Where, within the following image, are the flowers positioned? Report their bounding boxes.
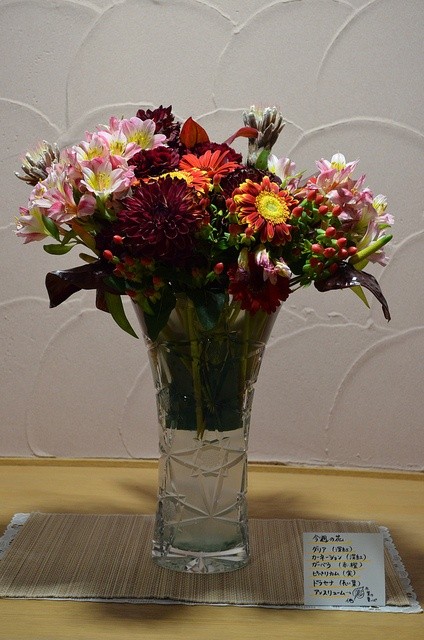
[14,104,393,432]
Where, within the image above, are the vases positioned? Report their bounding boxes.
[126,288,293,573]
